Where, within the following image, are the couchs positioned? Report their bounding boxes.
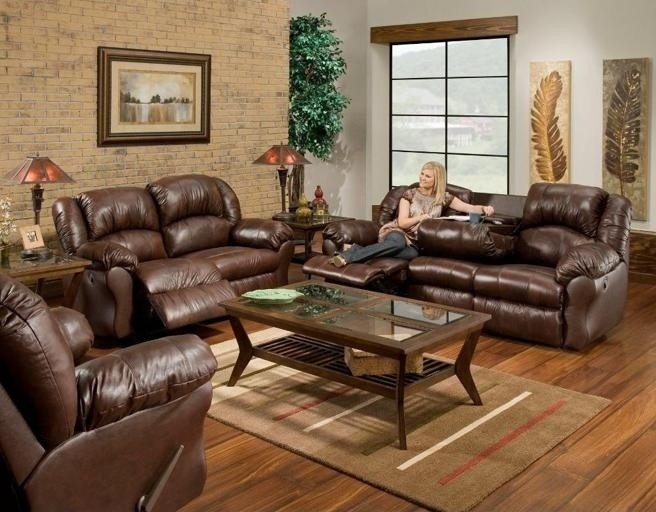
[50,173,297,343]
[301,180,633,354]
[0,272,219,512]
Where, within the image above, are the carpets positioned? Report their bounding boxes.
[204,325,615,511]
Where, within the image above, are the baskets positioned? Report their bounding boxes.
[344,334,424,376]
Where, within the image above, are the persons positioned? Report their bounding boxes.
[329,160,496,268]
[30,231,38,241]
[27,232,32,240]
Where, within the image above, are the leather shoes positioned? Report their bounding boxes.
[329,256,345,267]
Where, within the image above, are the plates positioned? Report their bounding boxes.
[240,287,306,305]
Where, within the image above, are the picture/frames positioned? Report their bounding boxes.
[96,45,212,147]
[20,224,45,250]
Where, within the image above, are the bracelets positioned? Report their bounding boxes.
[481,206,487,213]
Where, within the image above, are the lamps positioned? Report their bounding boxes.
[2,151,76,263]
[252,140,312,222]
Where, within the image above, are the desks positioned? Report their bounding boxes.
[289,215,355,265]
[0,249,93,298]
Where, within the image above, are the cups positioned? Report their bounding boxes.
[468,212,484,225]
